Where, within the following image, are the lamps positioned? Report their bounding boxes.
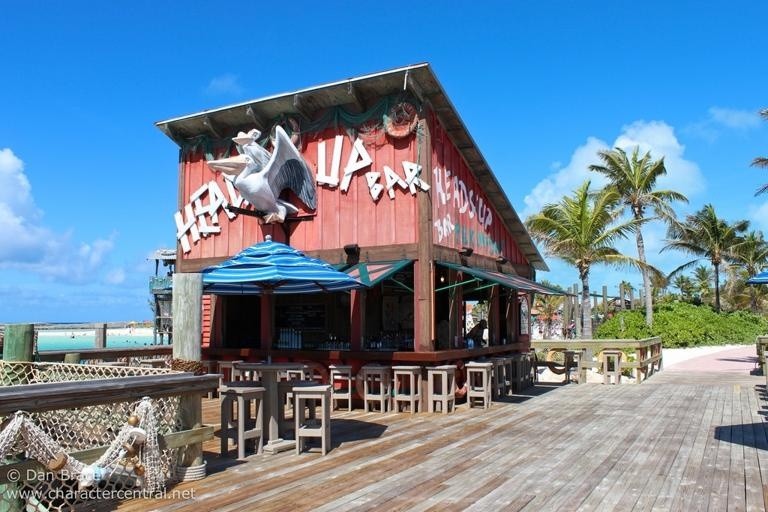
[459,247,507,265]
[344,243,360,257]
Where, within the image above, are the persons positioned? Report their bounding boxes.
[465,318,489,348]
[436,308,457,350]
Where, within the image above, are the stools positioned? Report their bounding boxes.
[97,350,622,460]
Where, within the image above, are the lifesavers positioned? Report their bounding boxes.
[298,362,329,406]
[454,360,467,399]
[546,348,573,374]
[599,348,627,375]
[383,102,418,137]
[356,363,399,402]
[270,116,301,147]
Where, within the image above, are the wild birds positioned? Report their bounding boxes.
[206,125,318,223]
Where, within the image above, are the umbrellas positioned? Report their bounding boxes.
[744,270,768,286]
[199,233,371,365]
